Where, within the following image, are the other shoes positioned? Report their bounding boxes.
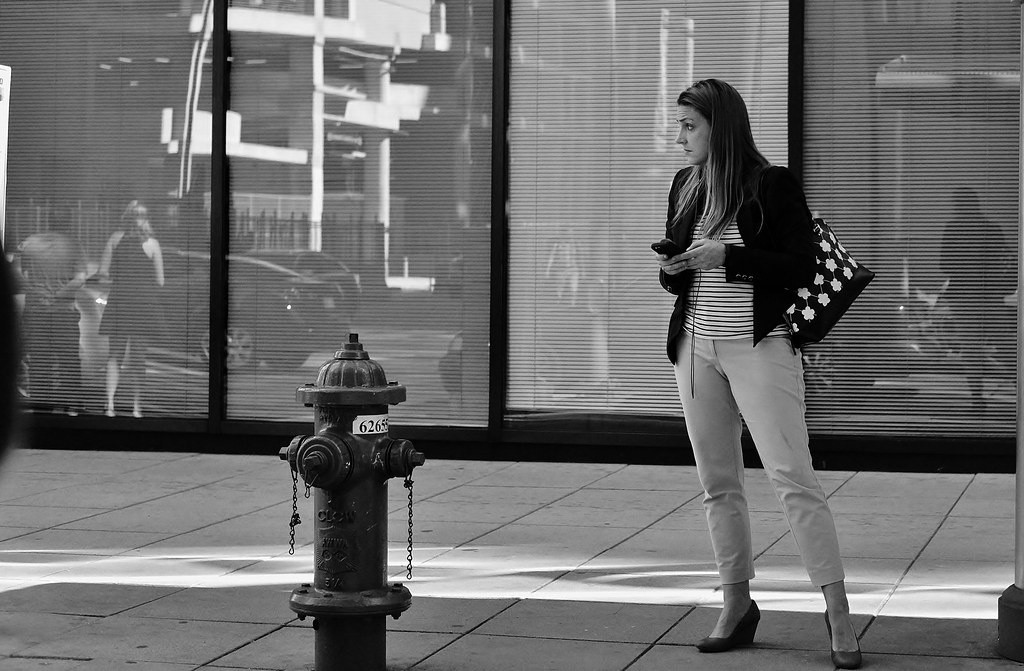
[105,411,116,416]
[964,406,987,420]
[133,411,144,417]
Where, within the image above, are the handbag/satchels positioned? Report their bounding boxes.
[1000,255,1018,296]
[782,219,875,347]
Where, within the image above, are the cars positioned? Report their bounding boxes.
[4,249,362,405]
[806,290,915,408]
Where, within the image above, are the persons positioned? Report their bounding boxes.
[545,226,585,307]
[97,200,165,417]
[940,186,1017,416]
[12,203,89,409]
[654,78,862,670]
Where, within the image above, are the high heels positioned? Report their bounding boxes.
[824,610,863,669]
[696,599,761,649]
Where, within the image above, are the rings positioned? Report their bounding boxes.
[661,267,665,272]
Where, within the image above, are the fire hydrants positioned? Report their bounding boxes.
[279,334,425,671]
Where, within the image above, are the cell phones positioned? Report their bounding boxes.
[650,241,686,258]
